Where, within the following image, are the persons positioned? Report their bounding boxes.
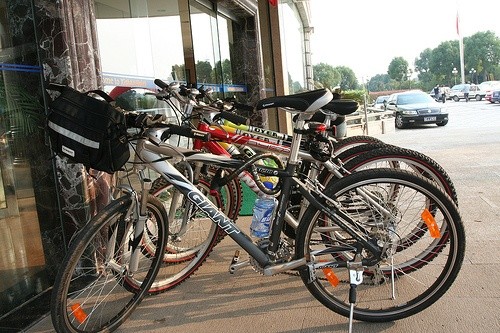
[461,81,469,102]
[440,85,447,103]
[434,84,440,102]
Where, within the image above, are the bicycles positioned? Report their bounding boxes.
[44,81,466,333]
[111,77,460,296]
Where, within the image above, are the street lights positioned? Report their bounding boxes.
[452,68,458,85]
[468,67,477,83]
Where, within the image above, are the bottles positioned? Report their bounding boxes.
[249,181,276,238]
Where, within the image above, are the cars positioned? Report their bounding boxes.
[448,81,500,104]
[430,86,452,101]
[386,91,448,129]
[373,93,399,117]
